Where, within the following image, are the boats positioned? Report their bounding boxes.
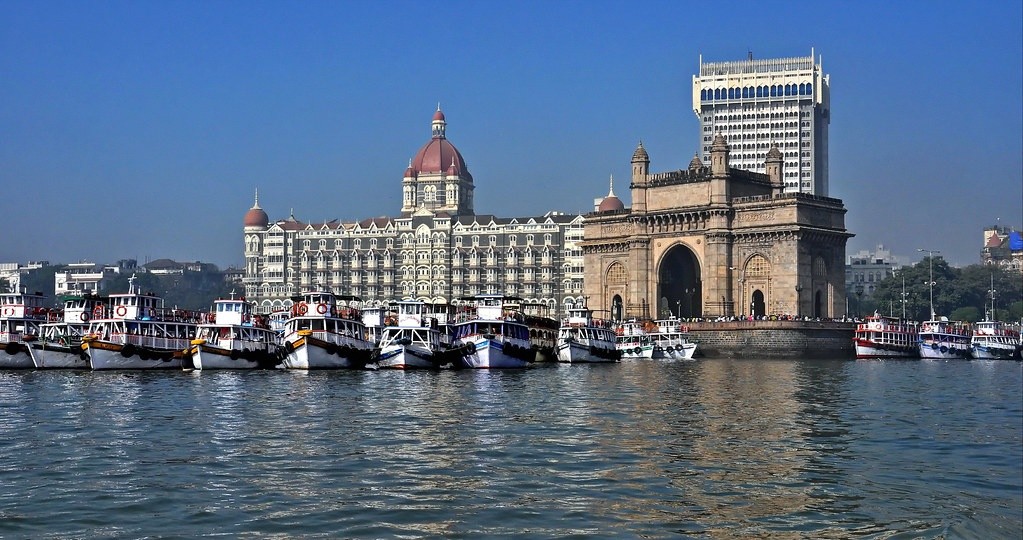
[851,276,919,359]
[276,292,375,370]
[970,273,1023,361]
[522,303,561,363]
[190,287,277,370]
[453,294,537,370]
[81,272,200,371]
[554,308,618,363]
[613,314,698,361]
[375,301,442,370]
[22,294,112,369]
[917,282,972,359]
[0,293,64,371]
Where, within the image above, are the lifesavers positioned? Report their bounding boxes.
[502,342,535,363]
[326,342,374,364]
[873,343,917,354]
[627,349,632,354]
[38,307,47,318]
[298,304,308,315]
[5,342,19,355]
[675,344,683,351]
[49,308,56,321]
[138,348,174,362]
[665,346,673,354]
[990,349,998,356]
[657,347,663,352]
[4,307,15,316]
[229,340,293,365]
[931,343,966,357]
[859,323,863,329]
[924,325,932,332]
[634,347,642,354]
[116,306,127,316]
[999,348,1013,356]
[434,341,476,366]
[330,305,337,316]
[24,308,35,319]
[875,323,882,330]
[317,303,328,314]
[121,343,136,358]
[589,346,624,361]
[80,312,90,321]
[242,313,251,324]
[93,306,103,316]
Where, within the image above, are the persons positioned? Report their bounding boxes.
[683,314,859,323]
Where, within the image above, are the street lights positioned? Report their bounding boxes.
[915,247,941,321]
[686,288,695,322]
